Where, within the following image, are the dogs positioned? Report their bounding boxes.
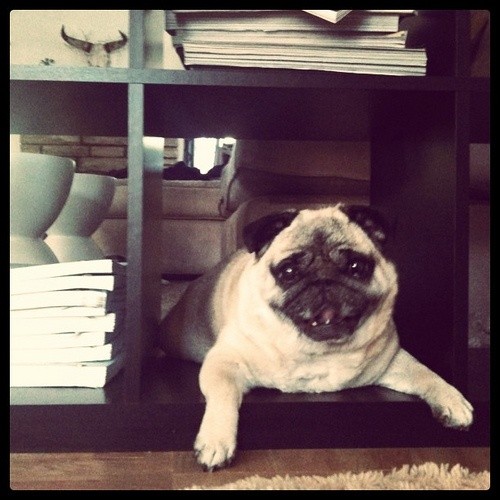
[156,203,473,471]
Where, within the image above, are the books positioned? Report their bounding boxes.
[163,10,431,77]
[8,257,127,391]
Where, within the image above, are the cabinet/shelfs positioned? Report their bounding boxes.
[10,7,490,452]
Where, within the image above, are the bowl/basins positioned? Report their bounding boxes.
[7,153,78,265]
[44,173,120,268]
